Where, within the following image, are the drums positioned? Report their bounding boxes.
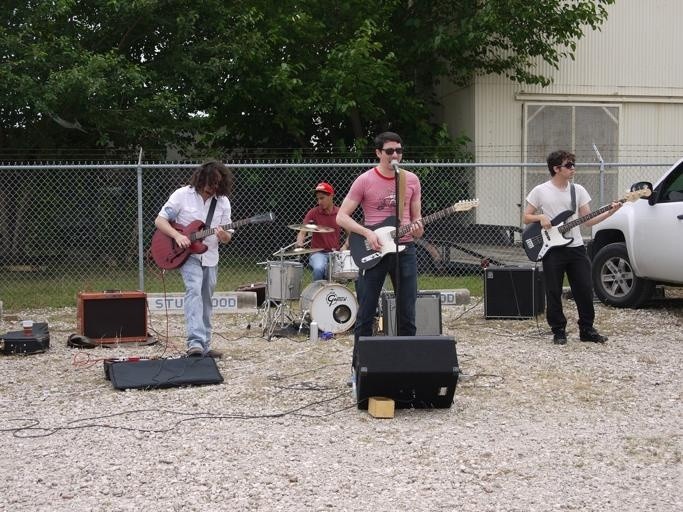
[264,260,303,301]
[299,280,357,335]
[326,251,361,280]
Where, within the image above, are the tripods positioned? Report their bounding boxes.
[263,249,309,341]
[248,271,297,328]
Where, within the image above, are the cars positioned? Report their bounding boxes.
[413,223,544,275]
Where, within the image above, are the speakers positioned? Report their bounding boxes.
[349,336,460,409]
[485,265,546,318]
[78,290,148,343]
[382,291,442,336]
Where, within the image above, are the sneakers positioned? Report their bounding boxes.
[187,347,223,357]
[555,330,567,344]
[581,332,608,343]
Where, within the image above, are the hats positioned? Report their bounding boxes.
[310,181,335,197]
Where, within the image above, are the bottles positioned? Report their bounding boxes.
[309,319,318,345]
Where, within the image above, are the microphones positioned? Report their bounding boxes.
[391,159,401,174]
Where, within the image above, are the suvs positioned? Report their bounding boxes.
[586,158,682,310]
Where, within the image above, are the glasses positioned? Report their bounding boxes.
[380,147,403,155]
[555,162,575,169]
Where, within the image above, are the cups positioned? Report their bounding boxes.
[22,320,33,336]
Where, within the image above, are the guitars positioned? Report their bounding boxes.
[523,185,652,261]
[151,212,275,270]
[349,199,480,270]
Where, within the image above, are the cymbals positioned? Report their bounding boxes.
[276,247,323,257]
[288,224,334,232]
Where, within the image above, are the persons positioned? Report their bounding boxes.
[294,181,356,282]
[334,130,426,375]
[520,150,623,347]
[153,160,236,358]
